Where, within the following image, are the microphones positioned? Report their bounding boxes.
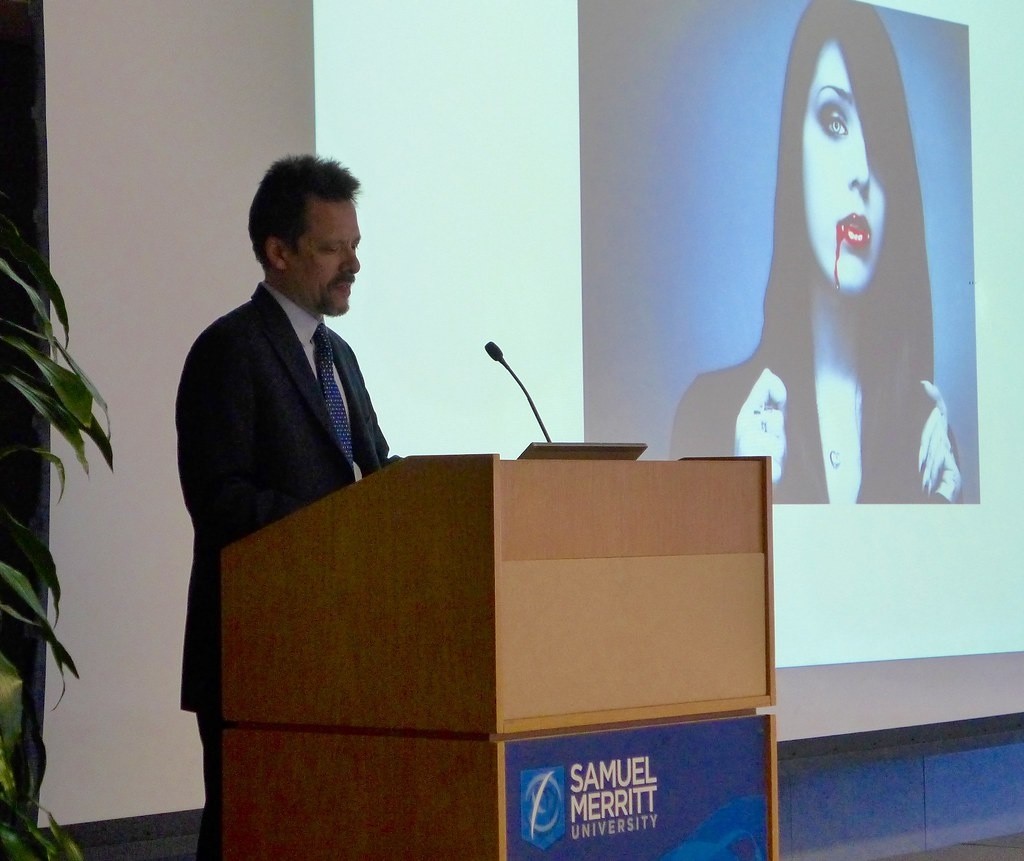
[485,341,552,443]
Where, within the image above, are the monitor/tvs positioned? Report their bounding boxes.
[515,443,646,460]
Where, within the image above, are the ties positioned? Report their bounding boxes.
[313,323,353,470]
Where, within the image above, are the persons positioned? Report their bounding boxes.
[668,1,974,504]
[171,154,400,859]
